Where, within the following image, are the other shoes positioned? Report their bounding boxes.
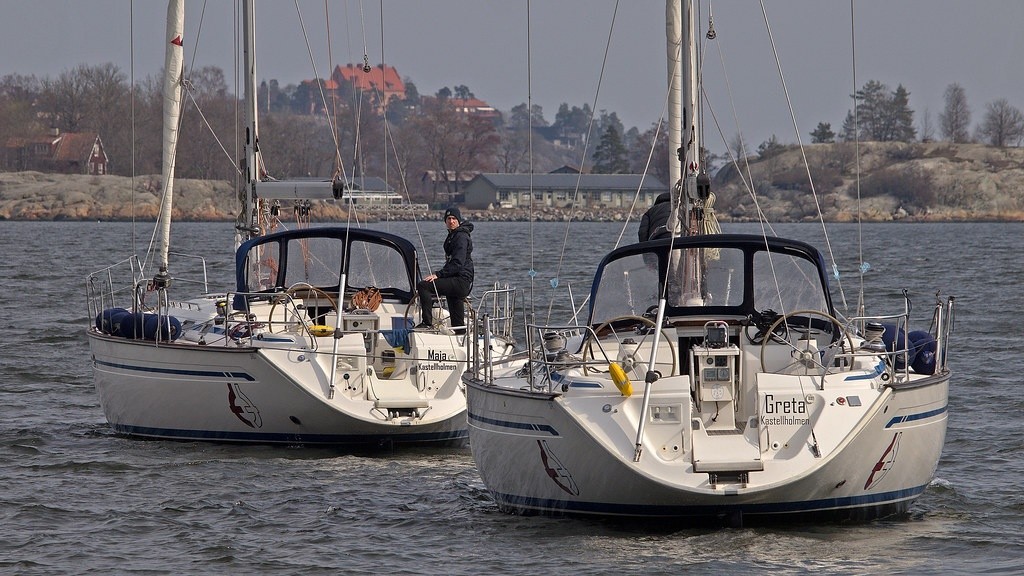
[415,322,433,329]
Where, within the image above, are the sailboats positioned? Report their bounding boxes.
[79,1,521,446]
[460,0,956,528]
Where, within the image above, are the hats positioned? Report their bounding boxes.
[444,209,461,224]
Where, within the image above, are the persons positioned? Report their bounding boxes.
[637,192,673,308]
[415,207,474,334]
